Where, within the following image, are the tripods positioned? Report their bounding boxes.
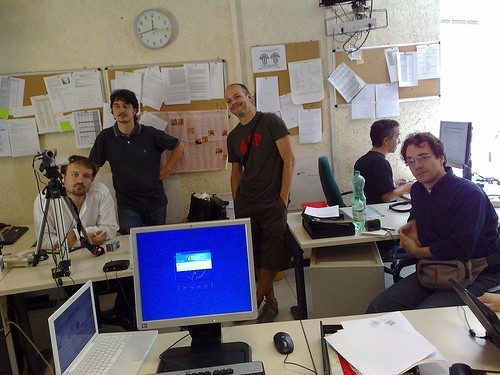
[33,167,99,276]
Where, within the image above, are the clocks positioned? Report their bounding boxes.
[134,9,173,49]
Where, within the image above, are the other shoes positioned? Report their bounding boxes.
[120,295,138,332]
[97,291,127,327]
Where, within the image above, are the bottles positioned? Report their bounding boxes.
[352,196,364,232]
[351,170,366,218]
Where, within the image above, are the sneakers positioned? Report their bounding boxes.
[232,294,265,325]
[258,298,280,324]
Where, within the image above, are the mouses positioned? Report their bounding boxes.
[274,332,294,354]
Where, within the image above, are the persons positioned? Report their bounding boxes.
[33,156,120,250]
[364,131,500,314]
[224,83,295,324]
[476,292,500,312]
[353,119,411,206]
[89,89,184,315]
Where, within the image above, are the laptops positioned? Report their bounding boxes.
[48,280,158,375]
[448,277,500,348]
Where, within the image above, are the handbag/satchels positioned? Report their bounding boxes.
[413,254,500,292]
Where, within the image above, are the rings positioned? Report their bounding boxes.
[94,233,97,236]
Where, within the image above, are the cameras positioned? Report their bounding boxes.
[130,218,258,330]
[440,121,472,168]
[38,147,70,171]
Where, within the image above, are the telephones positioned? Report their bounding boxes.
[3,248,39,269]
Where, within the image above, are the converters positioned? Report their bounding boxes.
[103,260,130,272]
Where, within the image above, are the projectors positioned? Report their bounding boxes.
[335,16,383,31]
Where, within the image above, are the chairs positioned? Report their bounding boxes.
[319,156,347,207]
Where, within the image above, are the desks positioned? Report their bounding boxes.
[138,306,500,375]
[0,225,36,280]
[0,235,134,375]
[287,202,412,320]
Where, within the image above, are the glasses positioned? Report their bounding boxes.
[401,151,435,168]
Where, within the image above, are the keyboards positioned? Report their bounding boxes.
[0,227,28,245]
[147,361,265,375]
